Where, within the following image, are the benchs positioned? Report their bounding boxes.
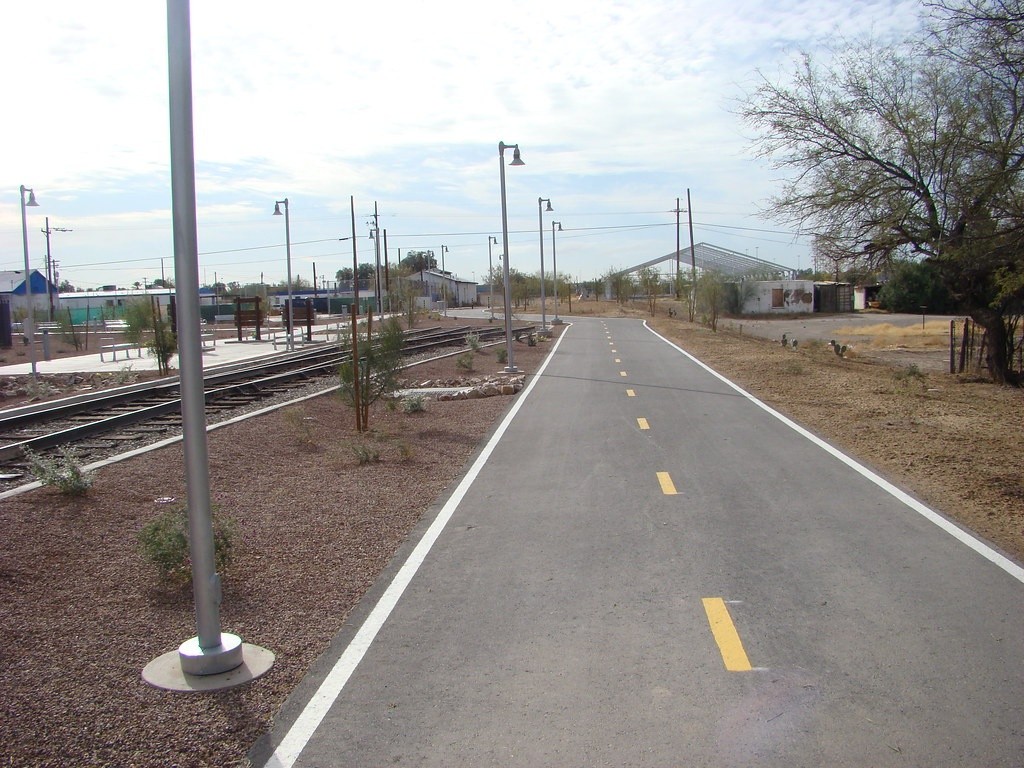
[35,322,63,332]
[104,320,131,334]
[99,338,142,363]
[198,323,215,346]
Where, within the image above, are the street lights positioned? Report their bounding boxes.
[442,244,450,319]
[368,227,385,323]
[551,221,564,321]
[497,140,525,373]
[426,250,435,300]
[538,196,554,330]
[488,235,499,320]
[19,183,40,376]
[272,197,295,347]
